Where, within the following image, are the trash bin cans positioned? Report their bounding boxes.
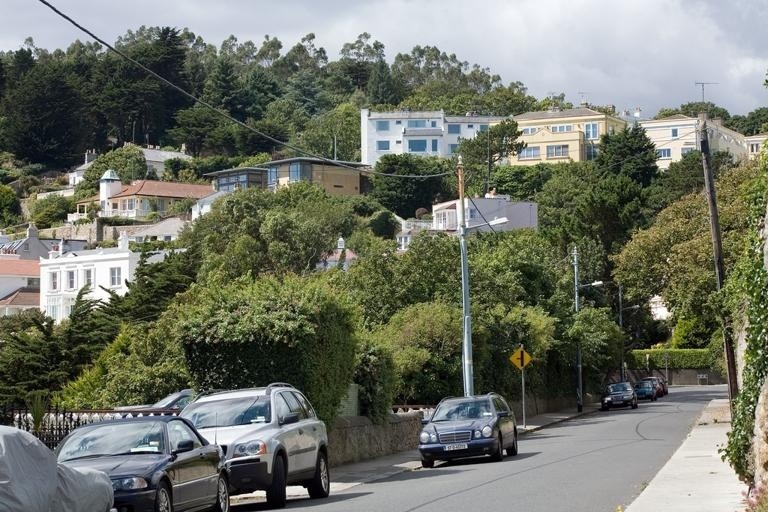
[697,374,708,385]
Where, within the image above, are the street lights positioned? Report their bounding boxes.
[619,305,641,381]
[461,214,511,398]
[575,280,603,412]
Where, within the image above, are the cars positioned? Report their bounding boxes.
[599,375,669,411]
[122,390,198,419]
[52,412,234,511]
[418,390,520,468]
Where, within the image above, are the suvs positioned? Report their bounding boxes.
[179,380,334,509]
